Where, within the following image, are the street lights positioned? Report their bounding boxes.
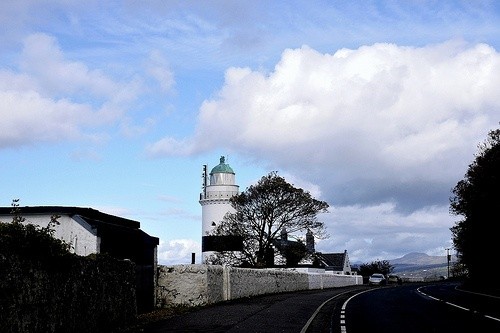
[446,248,452,280]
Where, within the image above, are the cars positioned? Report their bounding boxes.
[369,274,386,286]
[389,275,402,284]
